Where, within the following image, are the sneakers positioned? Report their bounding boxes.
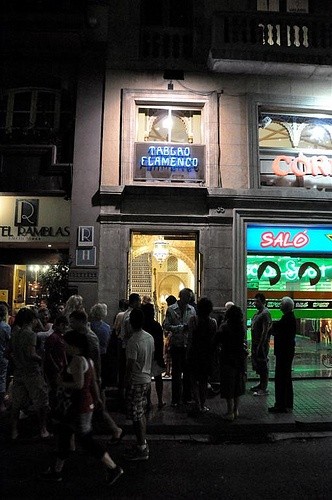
[104,464,124,486]
[128,446,138,455]
[127,447,149,460]
[42,468,63,481]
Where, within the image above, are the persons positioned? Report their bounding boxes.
[121,308,156,462]
[39,335,124,487]
[251,293,272,396]
[9,309,59,440]
[212,306,248,421]
[267,297,296,413]
[0,305,13,415]
[187,297,217,414]
[1,288,197,408]
[68,312,126,445]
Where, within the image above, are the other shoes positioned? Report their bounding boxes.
[40,432,54,441]
[253,386,270,395]
[158,403,167,409]
[220,411,240,421]
[105,429,127,448]
[146,402,153,409]
[170,403,177,407]
[200,406,210,412]
[250,382,265,391]
[268,405,292,413]
[187,397,196,404]
